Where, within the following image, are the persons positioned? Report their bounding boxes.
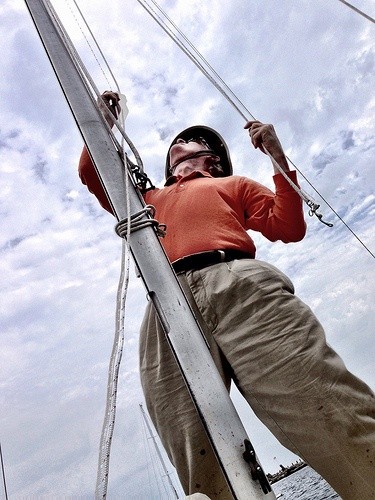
[77,88,375,500]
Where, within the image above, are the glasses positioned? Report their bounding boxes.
[184,137,211,151]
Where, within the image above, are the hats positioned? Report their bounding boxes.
[165,126,232,180]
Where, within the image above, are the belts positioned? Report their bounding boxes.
[173,250,251,274]
